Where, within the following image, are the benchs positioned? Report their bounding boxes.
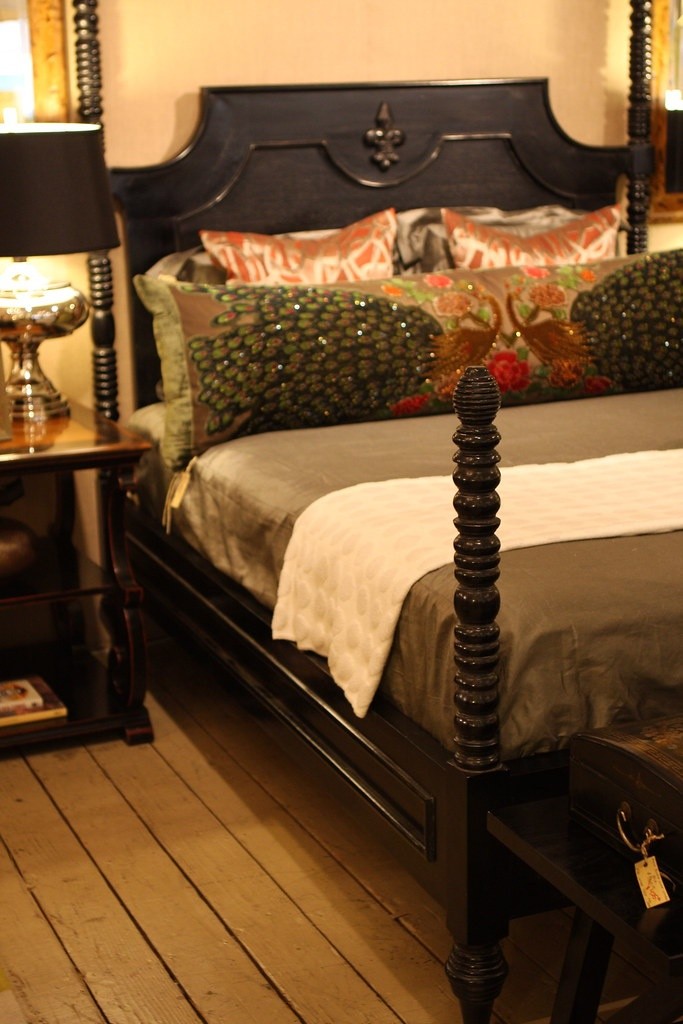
[486,796,683,1024]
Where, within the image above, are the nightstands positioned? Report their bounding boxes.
[0,400,154,751]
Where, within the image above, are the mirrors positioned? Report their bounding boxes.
[0,0,70,123]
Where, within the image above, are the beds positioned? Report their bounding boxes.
[103,74,683,1024]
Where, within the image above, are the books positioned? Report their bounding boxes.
[0,677,68,727]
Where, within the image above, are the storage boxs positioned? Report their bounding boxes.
[568,713,683,903]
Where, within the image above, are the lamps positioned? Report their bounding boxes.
[0,122,121,425]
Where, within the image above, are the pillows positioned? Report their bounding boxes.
[134,201,683,471]
